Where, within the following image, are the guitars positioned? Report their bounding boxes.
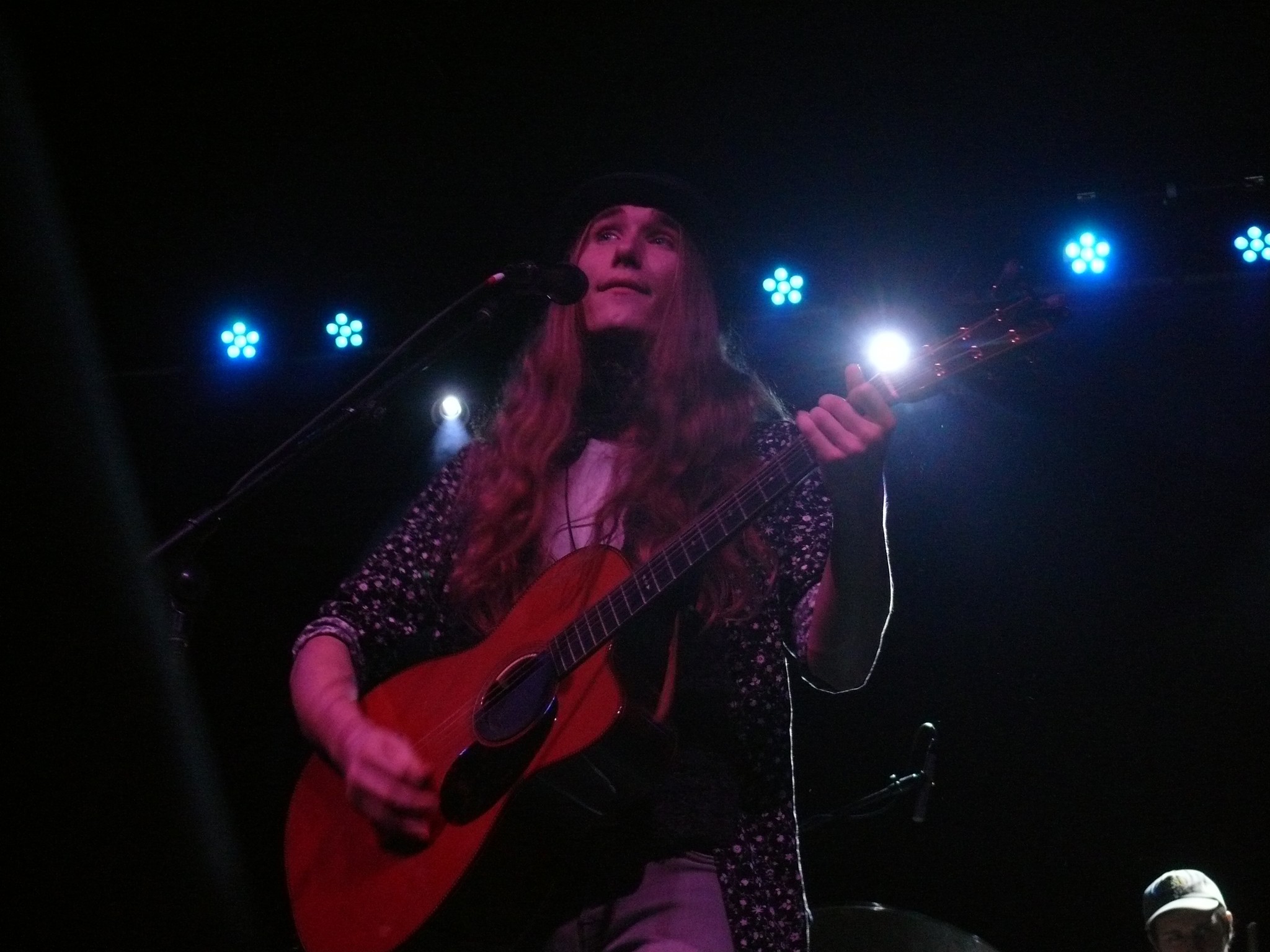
[277,289,1078,952]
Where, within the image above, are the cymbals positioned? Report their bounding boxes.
[802,901,993,952]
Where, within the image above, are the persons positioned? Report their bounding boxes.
[288,180,899,952]
[1144,869,1233,952]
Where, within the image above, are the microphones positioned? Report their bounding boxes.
[504,261,589,305]
[914,740,938,822]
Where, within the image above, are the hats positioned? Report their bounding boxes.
[1141,870,1228,930]
[572,170,708,236]
